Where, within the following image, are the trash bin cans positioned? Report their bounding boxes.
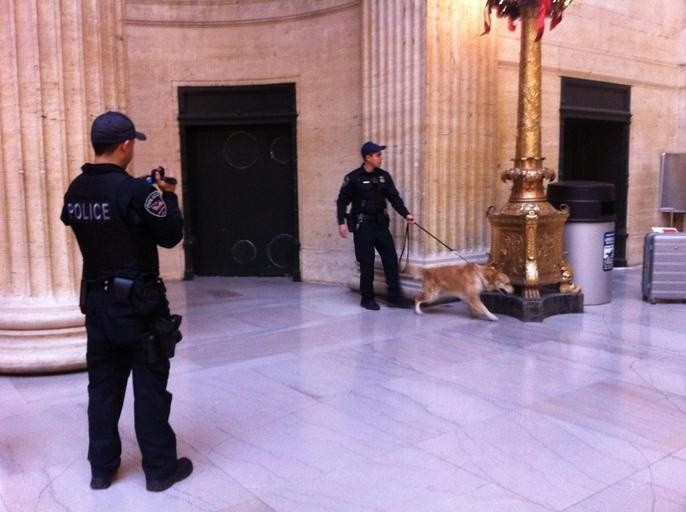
[547,181,617,305]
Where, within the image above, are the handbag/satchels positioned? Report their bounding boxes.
[112,278,134,306]
[346,214,356,232]
[79,278,96,315]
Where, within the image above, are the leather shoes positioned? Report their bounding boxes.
[90,465,119,489]
[388,298,410,308]
[361,297,380,310]
[144,457,193,491]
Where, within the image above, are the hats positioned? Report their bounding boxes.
[91,111,146,142]
[361,142,386,155]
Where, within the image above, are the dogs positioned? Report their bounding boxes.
[403,264,515,321]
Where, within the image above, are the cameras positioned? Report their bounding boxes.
[144,168,177,184]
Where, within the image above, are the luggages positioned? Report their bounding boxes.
[641,226,686,304]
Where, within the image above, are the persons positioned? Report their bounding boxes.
[333,139,417,312]
[55,108,193,493]
